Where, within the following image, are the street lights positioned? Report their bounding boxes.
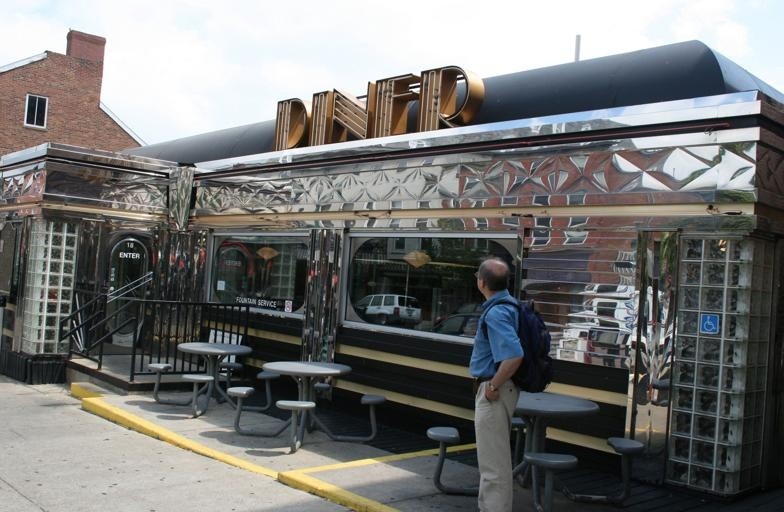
[404,250,432,314]
[255,246,280,307]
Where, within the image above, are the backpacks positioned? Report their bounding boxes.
[482,298,553,394]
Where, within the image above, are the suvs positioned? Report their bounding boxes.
[357,294,422,328]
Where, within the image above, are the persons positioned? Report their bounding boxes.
[466,257,526,511]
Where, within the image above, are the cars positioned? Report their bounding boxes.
[426,313,482,340]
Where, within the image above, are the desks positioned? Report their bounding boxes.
[176,342,253,419]
[513,390,600,512]
[263,361,353,451]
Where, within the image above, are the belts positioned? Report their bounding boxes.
[477,376,493,383]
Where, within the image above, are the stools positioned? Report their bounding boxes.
[275,383,386,451]
[426,417,645,511]
[181,362,280,437]
[147,363,172,403]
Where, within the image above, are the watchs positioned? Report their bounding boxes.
[486,382,499,393]
